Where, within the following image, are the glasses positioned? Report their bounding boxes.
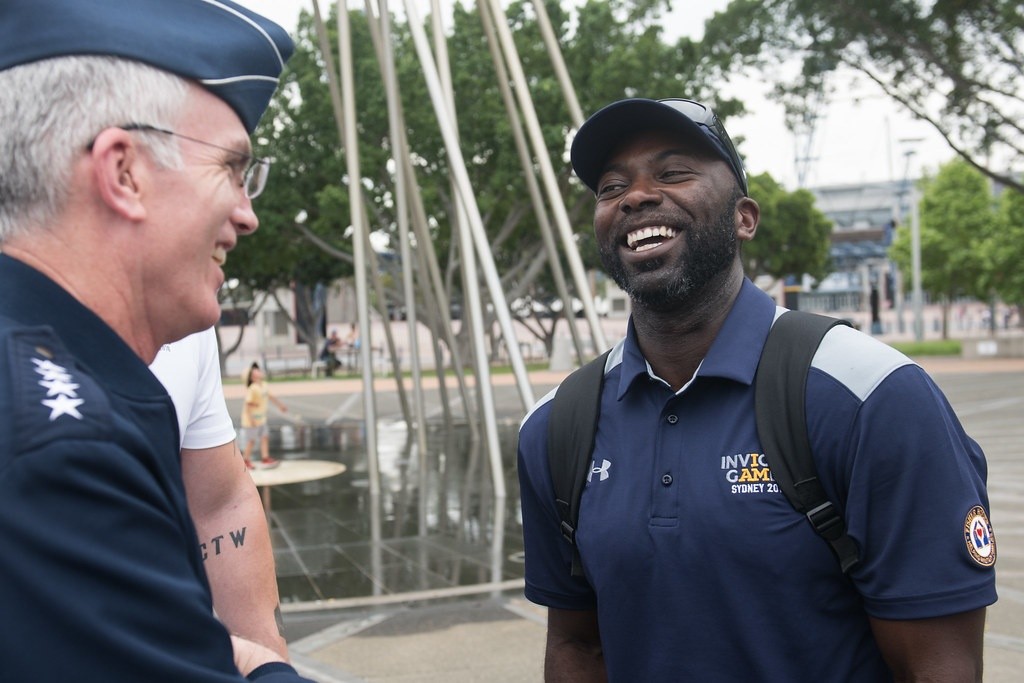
[86,125,271,199]
[657,97,749,198]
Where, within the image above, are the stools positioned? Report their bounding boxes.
[312,360,327,377]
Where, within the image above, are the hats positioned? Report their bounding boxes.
[0,0,297,134]
[570,98,747,196]
[240,362,259,386]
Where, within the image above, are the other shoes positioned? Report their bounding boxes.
[244,459,255,469]
[263,458,279,467]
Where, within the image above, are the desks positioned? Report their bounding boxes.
[334,347,386,378]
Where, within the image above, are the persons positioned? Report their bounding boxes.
[239,363,287,470]
[348,324,359,347]
[0,0,318,683]
[320,331,343,378]
[516,95,998,683]
[147,324,291,662]
